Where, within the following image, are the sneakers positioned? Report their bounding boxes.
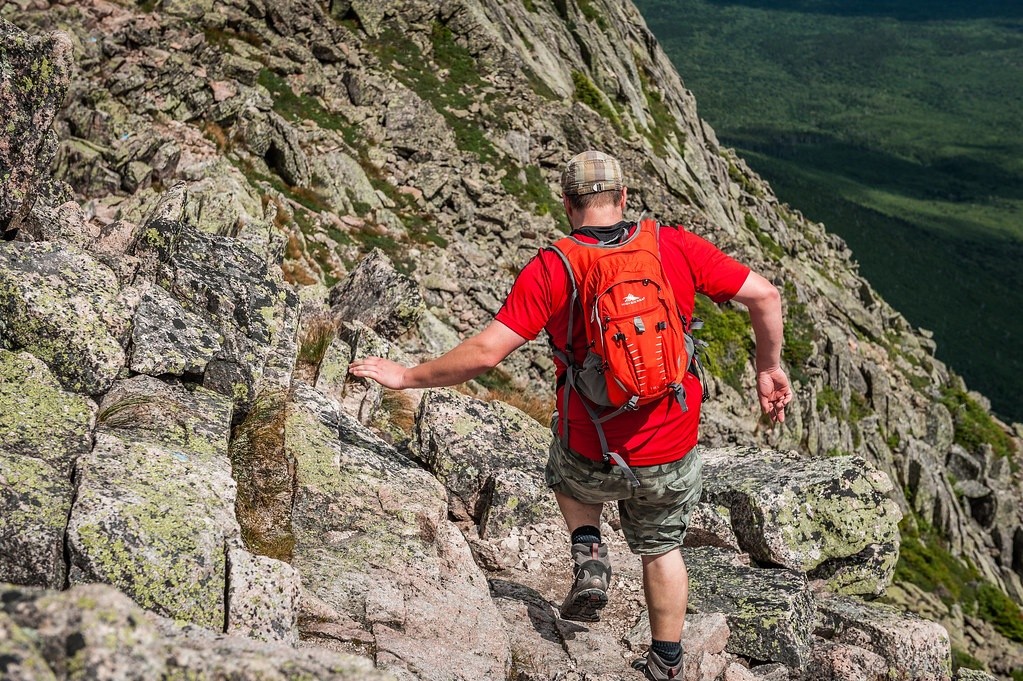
[631,645,683,681]
[559,542,612,622]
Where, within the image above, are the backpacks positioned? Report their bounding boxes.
[544,218,709,413]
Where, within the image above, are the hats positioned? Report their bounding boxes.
[560,151,623,195]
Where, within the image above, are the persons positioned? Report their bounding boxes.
[349,151,793,681]
